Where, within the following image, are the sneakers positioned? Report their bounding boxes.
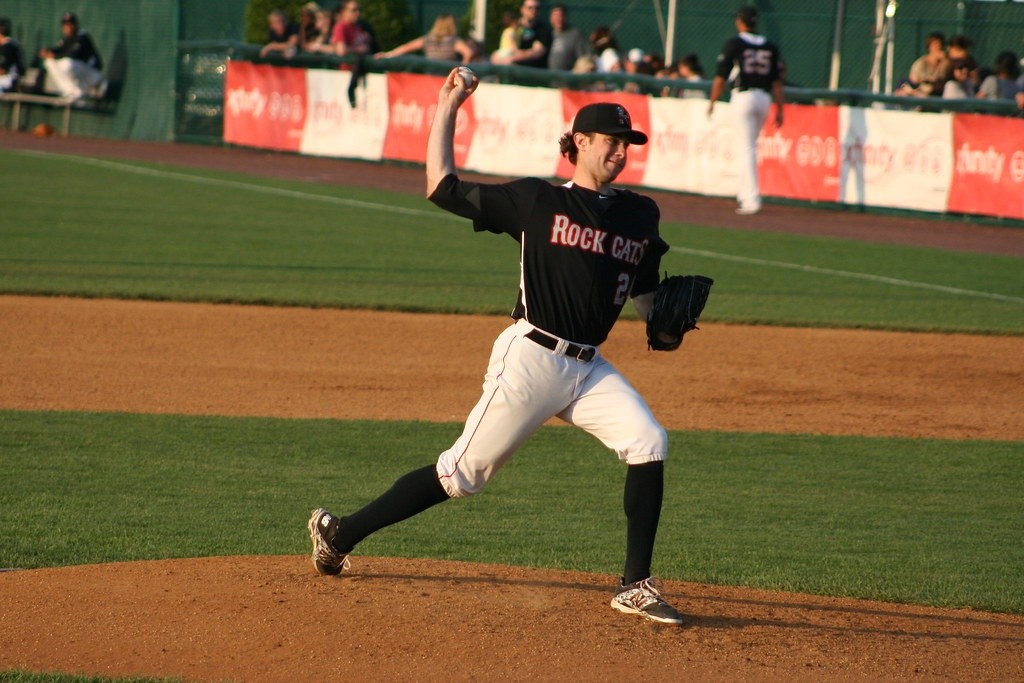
[611,576,685,624]
[308,508,351,576]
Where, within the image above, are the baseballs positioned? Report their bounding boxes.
[453,65,475,88]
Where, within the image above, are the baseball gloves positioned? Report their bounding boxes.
[646,275,714,350]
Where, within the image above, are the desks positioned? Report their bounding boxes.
[0,92,98,135]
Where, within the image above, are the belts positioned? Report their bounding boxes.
[525,329,595,363]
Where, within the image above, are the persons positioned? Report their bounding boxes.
[0,15,26,92]
[890,31,1024,121]
[707,6,785,216]
[259,0,711,81]
[40,12,104,107]
[306,66,715,623]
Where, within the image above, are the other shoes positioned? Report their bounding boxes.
[736,205,760,215]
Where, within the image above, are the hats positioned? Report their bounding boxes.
[572,103,647,146]
[59,12,79,25]
[628,48,645,62]
